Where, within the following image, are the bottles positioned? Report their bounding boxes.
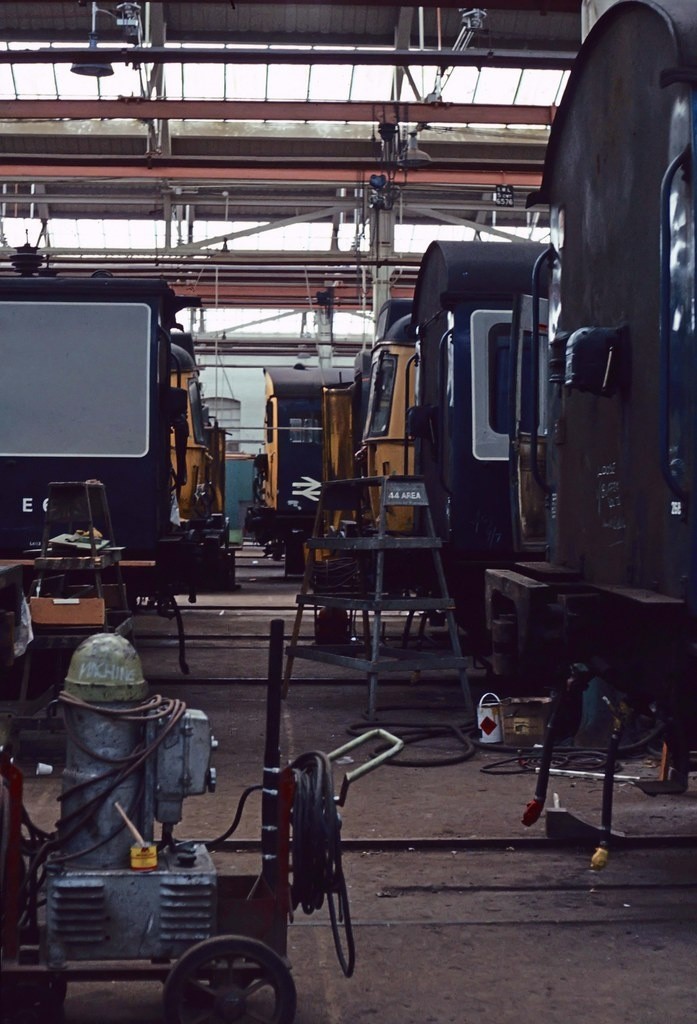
[130,843,157,872]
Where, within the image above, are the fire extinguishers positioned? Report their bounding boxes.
[1,743,26,961]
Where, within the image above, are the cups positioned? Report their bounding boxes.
[36,763,53,775]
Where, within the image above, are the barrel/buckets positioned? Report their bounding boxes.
[476,692,504,744]
[499,696,553,748]
